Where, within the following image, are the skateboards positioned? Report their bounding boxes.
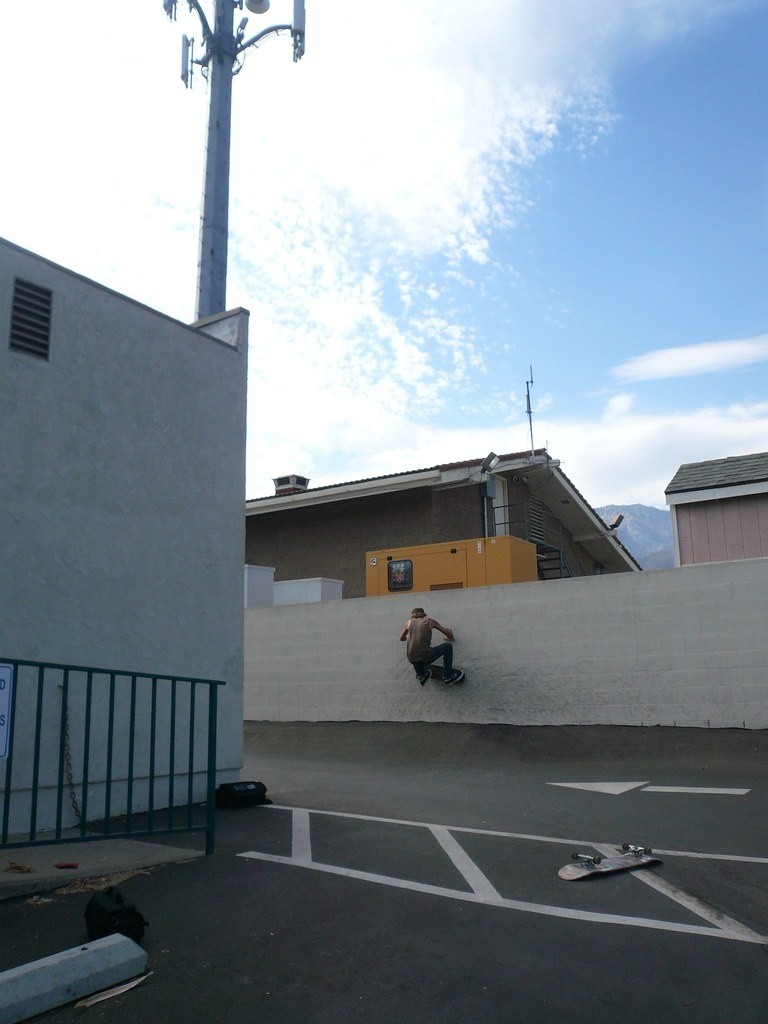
[558,842,663,883]
[425,664,466,687]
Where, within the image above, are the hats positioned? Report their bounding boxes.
[410,608,426,618]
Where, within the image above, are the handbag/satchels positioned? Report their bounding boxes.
[84,885,150,942]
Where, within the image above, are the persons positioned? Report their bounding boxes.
[400,607,464,688]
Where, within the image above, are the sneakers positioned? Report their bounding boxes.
[445,670,463,687]
[419,669,432,686]
